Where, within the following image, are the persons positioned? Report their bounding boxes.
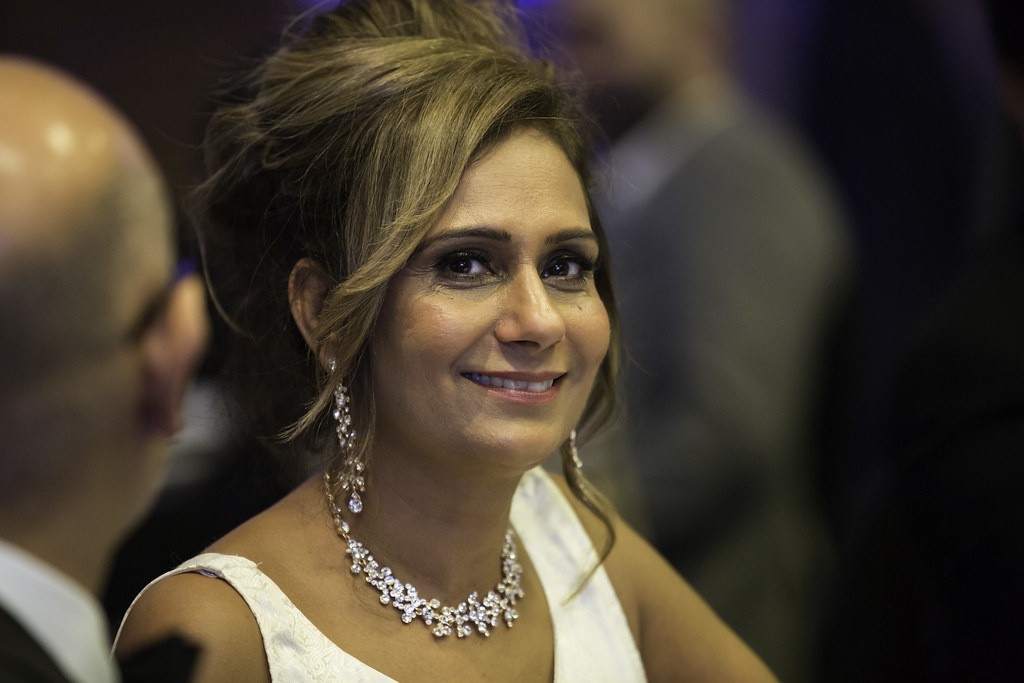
[110,0,782,683]
[550,0,849,683]
[0,54,209,683]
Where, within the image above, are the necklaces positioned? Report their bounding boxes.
[320,465,526,638]
